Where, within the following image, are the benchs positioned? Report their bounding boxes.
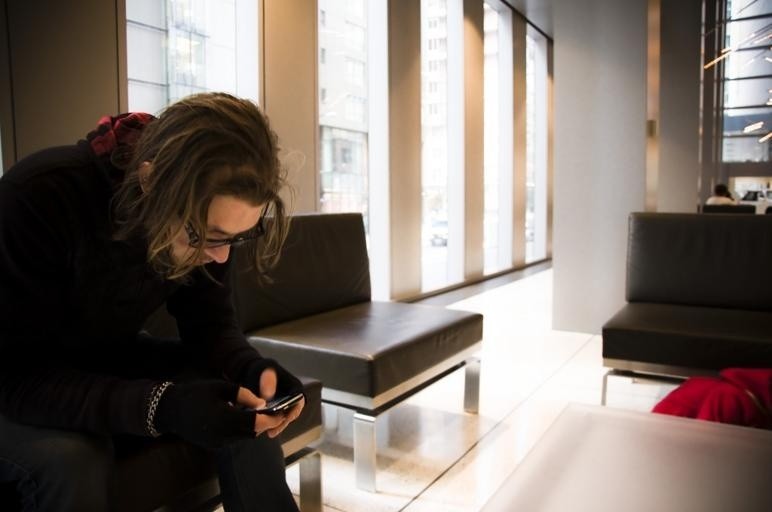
[207,212,483,493]
[765,206,772,214]
[197,371,327,512]
[702,205,756,213]
[600,211,772,409]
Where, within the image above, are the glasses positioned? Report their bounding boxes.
[178,207,265,249]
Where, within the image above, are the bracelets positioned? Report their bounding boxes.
[144,380,176,437]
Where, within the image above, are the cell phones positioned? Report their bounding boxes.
[243,391,305,415]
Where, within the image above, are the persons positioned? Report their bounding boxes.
[706,182,738,203]
[0,92,308,512]
